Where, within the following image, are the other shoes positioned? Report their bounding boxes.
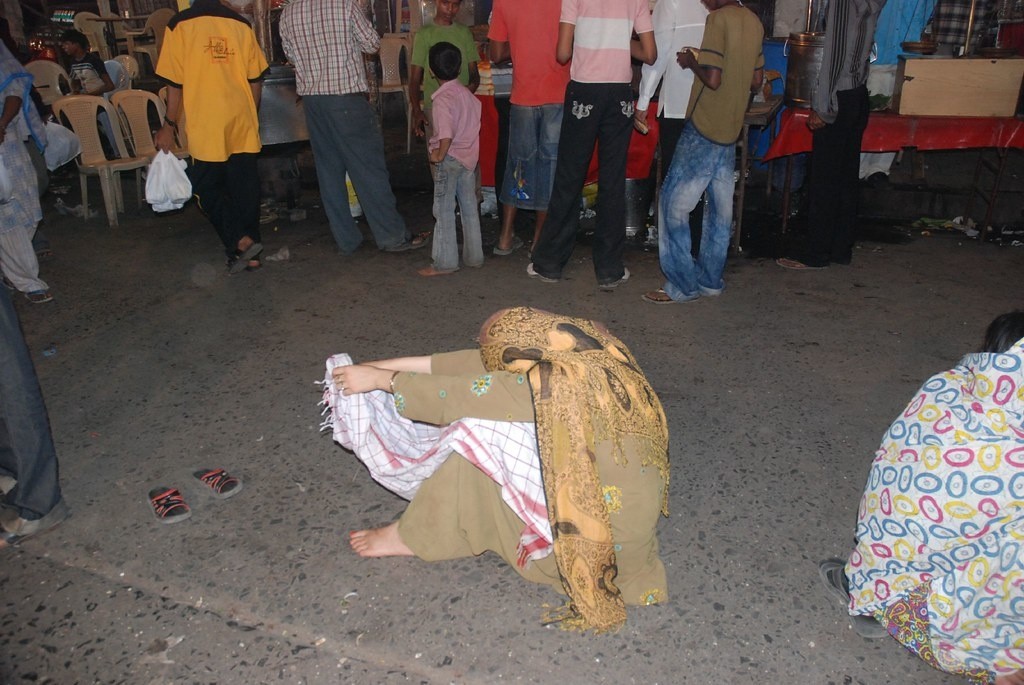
[225,242,265,272]
[527,264,559,282]
[601,266,631,291]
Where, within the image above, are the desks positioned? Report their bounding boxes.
[85,15,153,65]
[762,109,1024,233]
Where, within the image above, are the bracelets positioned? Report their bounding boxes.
[164,114,177,130]
[390,371,400,391]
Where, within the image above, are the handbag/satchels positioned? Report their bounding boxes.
[145,148,192,213]
[42,120,82,171]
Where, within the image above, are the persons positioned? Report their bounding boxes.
[820,310,1024,685]
[416,41,484,276]
[313,305,672,635]
[154,0,271,277]
[0,0,994,556]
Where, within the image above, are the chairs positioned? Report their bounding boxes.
[24,8,178,229]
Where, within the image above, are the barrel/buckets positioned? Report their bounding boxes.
[624,159,656,236]
[748,36,806,192]
[784,32,825,108]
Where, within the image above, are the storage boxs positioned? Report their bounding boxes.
[893,52,1024,117]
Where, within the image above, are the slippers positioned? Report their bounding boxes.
[192,468,242,500]
[493,236,524,255]
[24,288,54,304]
[384,235,430,251]
[817,557,892,639]
[147,487,192,525]
[0,513,71,553]
[776,256,831,271]
[0,274,17,290]
[642,288,703,303]
